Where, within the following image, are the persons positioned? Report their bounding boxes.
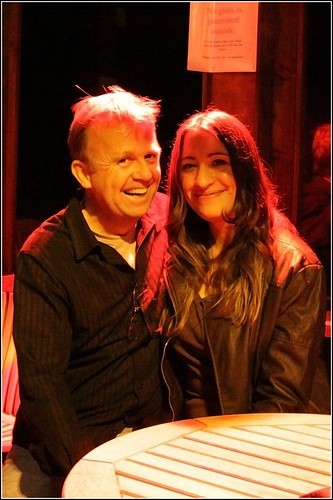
[1,84,182,500]
[165,109,333,420]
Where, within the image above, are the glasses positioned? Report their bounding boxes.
[125,286,145,341]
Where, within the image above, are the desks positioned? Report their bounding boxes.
[61,411,332,499]
